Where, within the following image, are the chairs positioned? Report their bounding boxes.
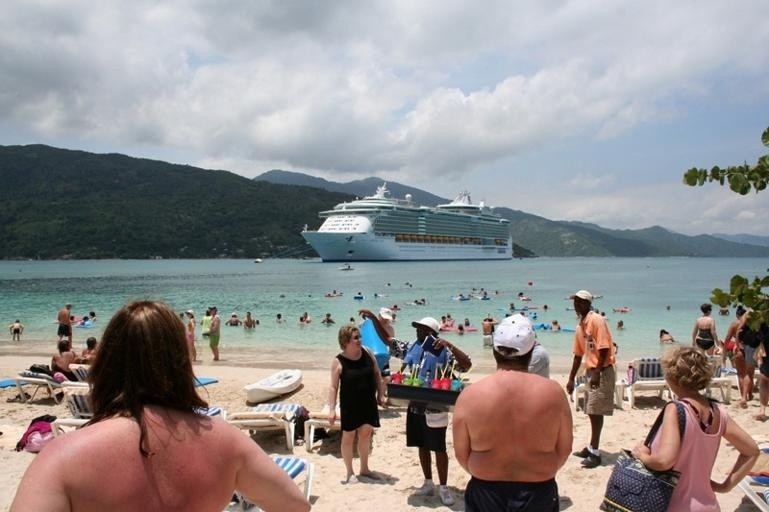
[58,379,98,419]
[9,368,58,408]
[720,365,761,393]
[301,402,348,455]
[225,452,314,512]
[65,359,98,382]
[617,351,680,413]
[697,354,735,408]
[224,399,307,453]
[191,401,230,421]
[568,371,626,416]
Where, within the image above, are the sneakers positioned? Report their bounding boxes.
[438,485,455,506]
[414,483,436,497]
[580,453,602,467]
[572,447,590,458]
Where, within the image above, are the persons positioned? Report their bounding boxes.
[618,320,623,329]
[6,299,313,511]
[692,303,769,421]
[321,314,336,324]
[325,290,616,512]
[388,282,413,287]
[632,346,761,511]
[614,306,632,313]
[528,281,533,286]
[472,287,484,293]
[660,330,675,343]
[667,305,671,310]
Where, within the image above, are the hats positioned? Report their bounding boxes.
[570,290,593,303]
[185,309,194,319]
[411,316,440,334]
[492,312,536,357]
[379,307,394,321]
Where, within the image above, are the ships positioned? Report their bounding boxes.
[298,179,513,263]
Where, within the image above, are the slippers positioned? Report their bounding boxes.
[340,473,355,485]
[360,471,381,480]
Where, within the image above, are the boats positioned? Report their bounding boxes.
[246,366,302,404]
[254,258,263,263]
[337,265,354,271]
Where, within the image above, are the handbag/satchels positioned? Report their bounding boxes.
[424,411,450,428]
[600,400,687,512]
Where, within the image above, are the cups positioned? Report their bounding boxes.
[392,373,462,391]
